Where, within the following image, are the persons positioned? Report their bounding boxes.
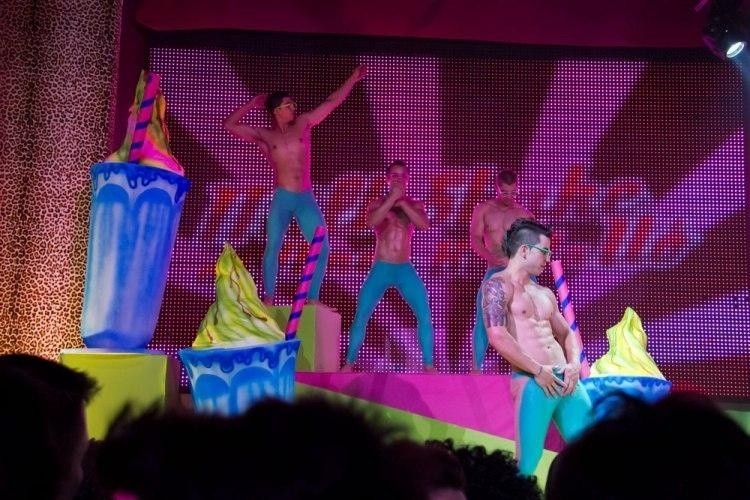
[223,62,368,306]
[470,172,535,373]
[338,157,446,374]
[479,218,593,475]
[0,352,749,500]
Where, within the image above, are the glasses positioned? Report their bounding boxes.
[523,242,553,260]
[276,101,297,112]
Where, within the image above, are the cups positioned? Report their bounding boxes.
[578,375,672,420]
[78,159,190,351]
[175,337,303,419]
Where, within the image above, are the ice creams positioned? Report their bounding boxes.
[581,307,673,422]
[81,67,189,350]
[178,240,300,418]
